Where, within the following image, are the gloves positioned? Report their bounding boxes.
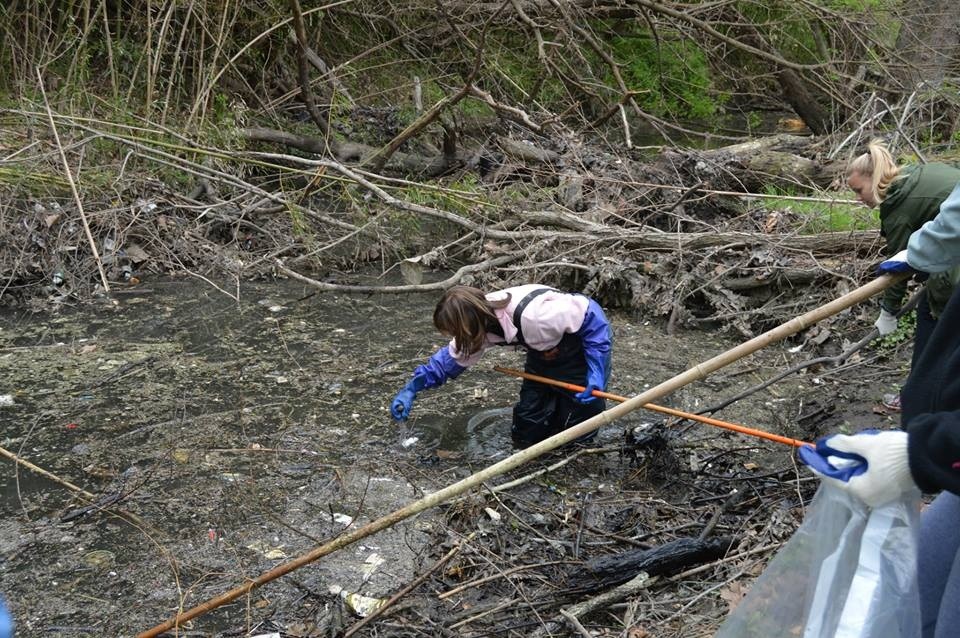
[800,429,917,509]
[874,309,897,335]
[573,299,611,404]
[877,249,930,283]
[391,346,467,419]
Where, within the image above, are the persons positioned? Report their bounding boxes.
[876,180,960,429]
[844,138,960,410]
[797,411,959,637]
[391,283,613,445]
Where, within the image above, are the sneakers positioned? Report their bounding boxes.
[882,393,902,409]
